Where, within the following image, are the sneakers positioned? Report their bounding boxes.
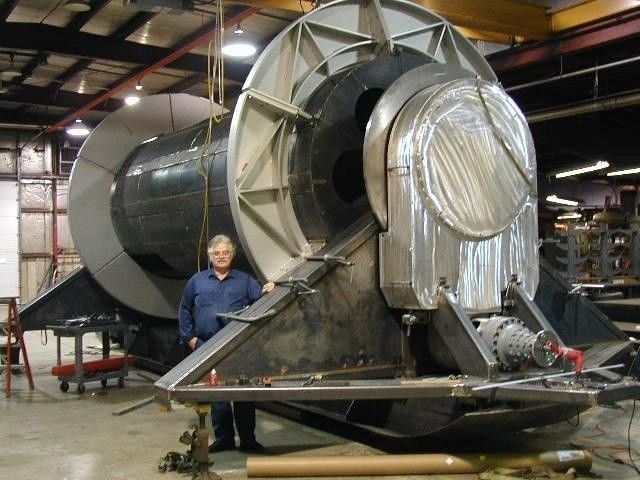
[240,441,265,453]
[208,438,235,452]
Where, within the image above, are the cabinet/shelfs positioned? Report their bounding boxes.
[45,325,128,394]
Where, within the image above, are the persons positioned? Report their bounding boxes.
[176,231,278,456]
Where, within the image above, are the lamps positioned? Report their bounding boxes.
[222,23,256,57]
[544,192,586,205]
[547,158,610,180]
[67,118,89,135]
[124,80,145,104]
[556,211,583,220]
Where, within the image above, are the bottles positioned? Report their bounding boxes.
[210,369,217,386]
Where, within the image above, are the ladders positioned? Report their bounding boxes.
[0,297,34,398]
